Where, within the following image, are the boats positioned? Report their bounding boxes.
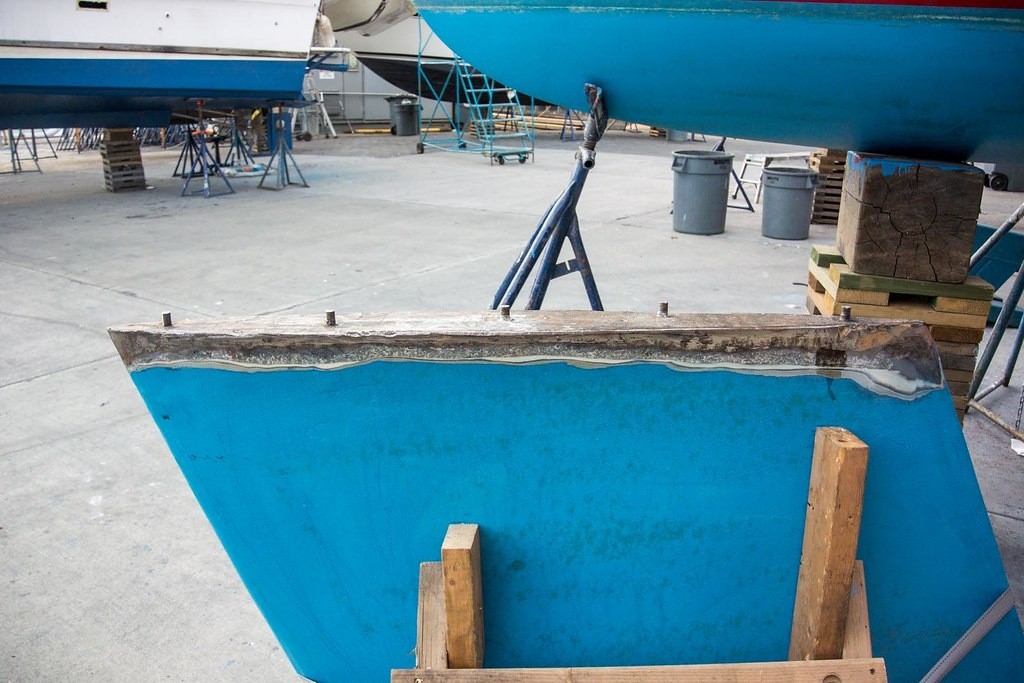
[406,1,1024,166]
[0,1,356,131]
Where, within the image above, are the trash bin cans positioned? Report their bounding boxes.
[671,149,736,235]
[761,167,822,239]
[393,103,422,135]
[267,112,293,150]
[384,96,418,133]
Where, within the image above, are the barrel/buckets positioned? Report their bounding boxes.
[670,150,735,234]
[760,167,820,240]
